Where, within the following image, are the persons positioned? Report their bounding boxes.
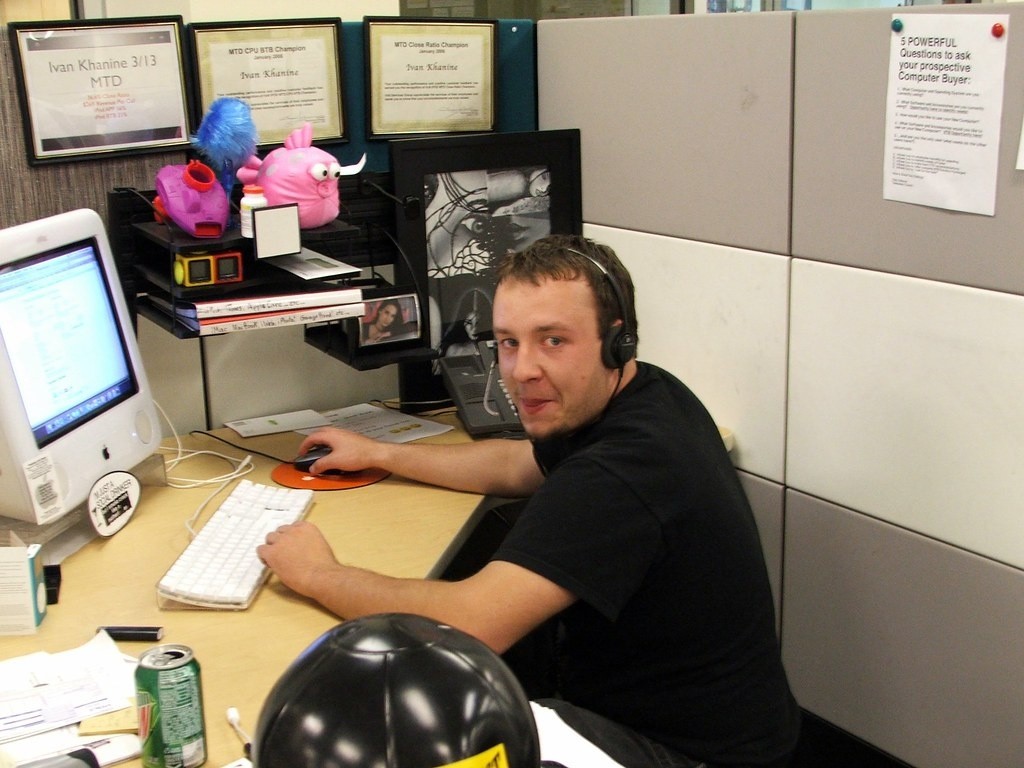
[256,235,800,768]
[440,290,494,357]
[361,299,418,344]
[423,169,493,278]
[486,166,550,202]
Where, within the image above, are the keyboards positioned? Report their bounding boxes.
[155,479,315,609]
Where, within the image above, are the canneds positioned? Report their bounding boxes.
[134,645,207,768]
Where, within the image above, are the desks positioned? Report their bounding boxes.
[0,396,734,768]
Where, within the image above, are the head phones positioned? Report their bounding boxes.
[568,251,637,369]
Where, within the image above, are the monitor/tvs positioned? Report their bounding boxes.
[0,208,162,526]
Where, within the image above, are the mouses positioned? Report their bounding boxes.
[293,447,359,475]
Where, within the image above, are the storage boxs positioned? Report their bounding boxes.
[0,543,48,635]
